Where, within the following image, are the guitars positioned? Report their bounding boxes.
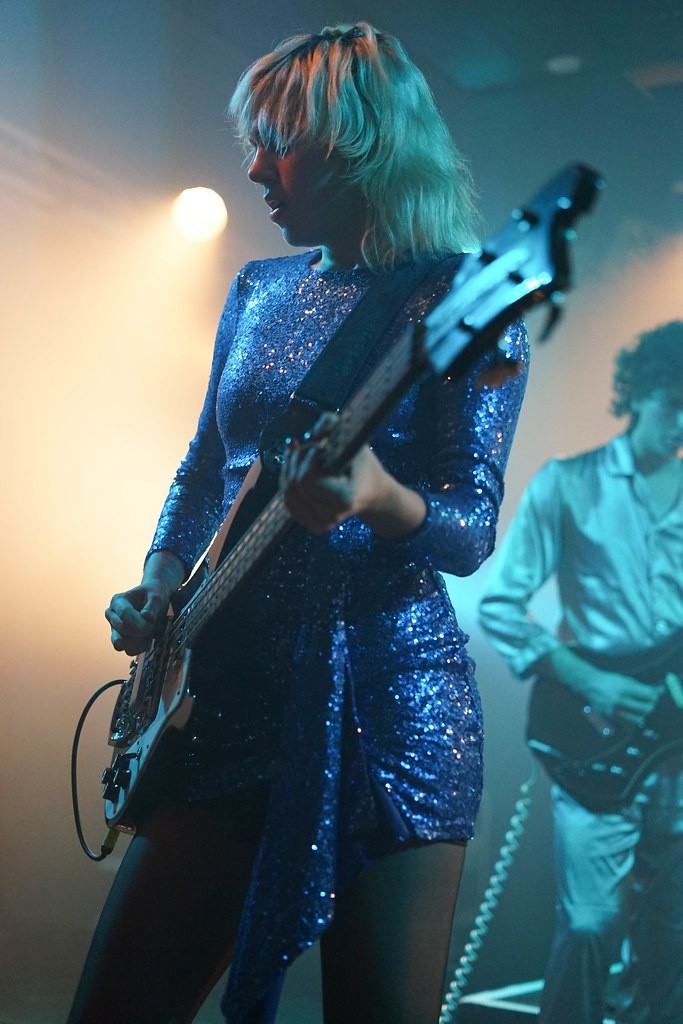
[100,160,602,831]
[526,625,682,816]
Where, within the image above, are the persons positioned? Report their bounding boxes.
[477,319,682,1024]
[68,23,529,1022]
